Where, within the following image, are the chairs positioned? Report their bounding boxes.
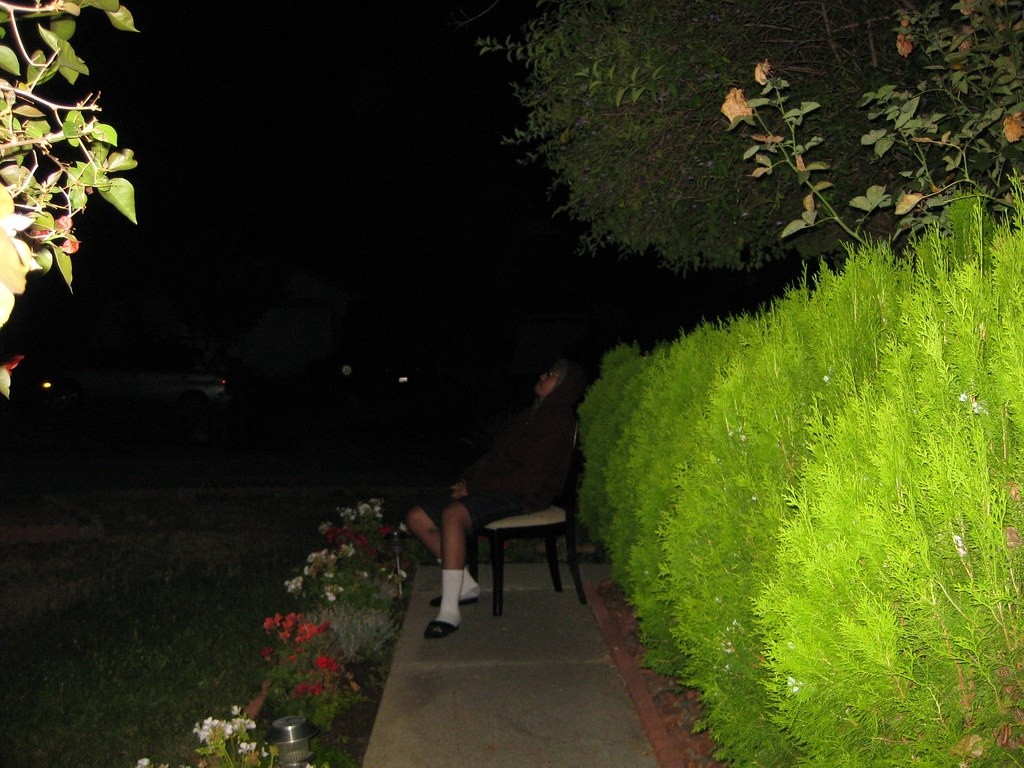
[466,419,588,616]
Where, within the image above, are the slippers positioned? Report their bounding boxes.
[424,621,459,639]
[430,596,479,607]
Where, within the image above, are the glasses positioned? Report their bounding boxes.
[543,369,560,377]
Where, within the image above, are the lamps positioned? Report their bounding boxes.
[382,524,411,600]
[263,716,321,768]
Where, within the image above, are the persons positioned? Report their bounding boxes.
[406,359,592,639]
